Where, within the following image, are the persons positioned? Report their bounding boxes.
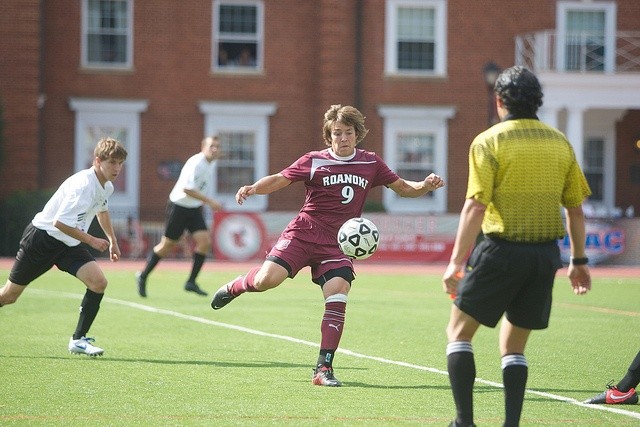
[211,104,445,386]
[583,347,639,405]
[441,66,592,426]
[135,136,227,297]
[0,137,129,356]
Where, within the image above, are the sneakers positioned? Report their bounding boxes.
[185,279,207,295]
[583,386,638,404]
[136,273,147,297]
[211,274,245,310]
[312,365,342,387]
[68,337,104,357]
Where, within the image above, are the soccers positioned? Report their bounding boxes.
[337,217,379,260]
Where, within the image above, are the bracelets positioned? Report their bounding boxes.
[569,257,588,267]
